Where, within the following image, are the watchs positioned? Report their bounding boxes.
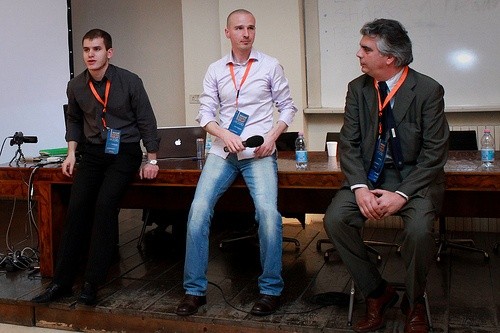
[146,160,159,166]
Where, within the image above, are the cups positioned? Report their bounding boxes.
[196,138,204,159]
[327,142,338,156]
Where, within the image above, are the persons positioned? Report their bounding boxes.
[177,9,298,316]
[322,18,451,333]
[30,29,161,307]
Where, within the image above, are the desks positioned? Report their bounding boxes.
[0,150,500,278]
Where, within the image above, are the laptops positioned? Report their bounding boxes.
[155,125,207,158]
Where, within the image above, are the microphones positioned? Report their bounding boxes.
[8,136,38,144]
[224,135,264,152]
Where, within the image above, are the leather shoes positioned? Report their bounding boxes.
[79,281,98,305]
[404,298,430,333]
[176,294,207,315]
[31,286,73,304]
[352,283,400,333]
[251,294,281,316]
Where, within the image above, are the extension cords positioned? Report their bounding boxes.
[6,257,32,272]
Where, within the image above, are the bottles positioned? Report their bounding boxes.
[481,129,494,168]
[295,132,308,168]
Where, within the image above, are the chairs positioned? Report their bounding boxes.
[318,132,403,264]
[435,131,490,262]
[220,133,301,250]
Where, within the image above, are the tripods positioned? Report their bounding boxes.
[8,143,26,168]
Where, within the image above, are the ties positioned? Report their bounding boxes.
[377,80,405,171]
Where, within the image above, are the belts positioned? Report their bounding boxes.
[383,163,396,170]
